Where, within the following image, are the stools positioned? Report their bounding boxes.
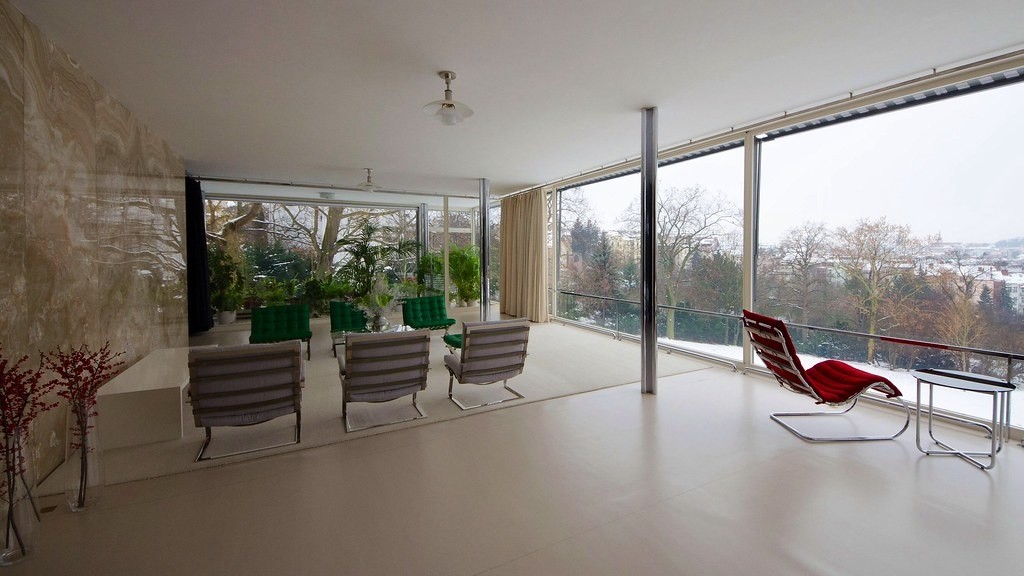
[444,334,462,352]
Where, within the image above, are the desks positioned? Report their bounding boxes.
[92,344,218,451]
[910,369,1017,470]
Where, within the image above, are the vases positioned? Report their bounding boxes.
[63,402,104,513]
[365,316,390,333]
[0,433,41,567]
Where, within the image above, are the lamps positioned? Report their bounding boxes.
[358,168,382,193]
[422,71,475,126]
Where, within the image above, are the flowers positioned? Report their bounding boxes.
[0,341,128,556]
[345,279,403,317]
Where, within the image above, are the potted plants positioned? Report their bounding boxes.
[211,292,244,326]
[432,246,481,307]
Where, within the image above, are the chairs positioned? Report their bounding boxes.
[338,328,430,433]
[188,339,306,464]
[249,306,312,362]
[330,300,381,358]
[742,310,911,444]
[401,294,455,336]
[443,317,530,412]
